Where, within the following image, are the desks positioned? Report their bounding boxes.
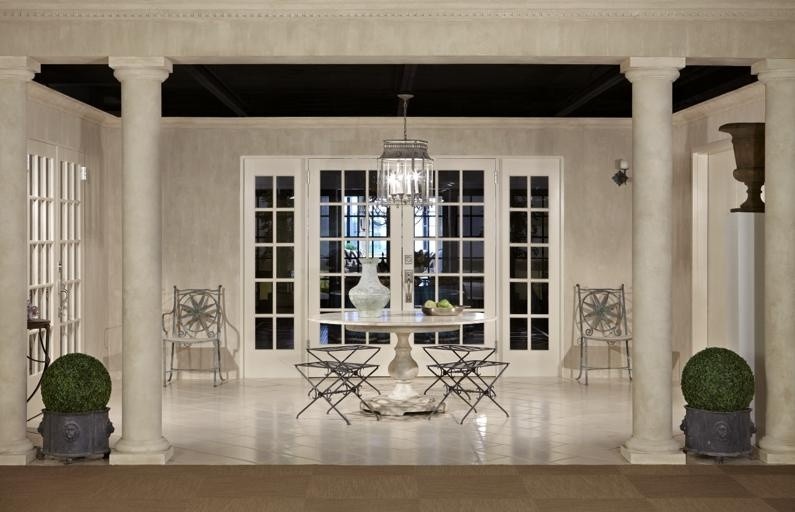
[26,318,52,423]
[307,305,500,417]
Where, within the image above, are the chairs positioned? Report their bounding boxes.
[159,283,230,387]
[570,282,632,387]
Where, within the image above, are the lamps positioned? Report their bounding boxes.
[611,159,630,186]
[373,92,434,205]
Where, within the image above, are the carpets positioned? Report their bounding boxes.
[0,462,794,511]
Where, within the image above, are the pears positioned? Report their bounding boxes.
[424,300,437,308]
[437,299,450,308]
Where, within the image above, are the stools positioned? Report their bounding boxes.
[296,343,511,427]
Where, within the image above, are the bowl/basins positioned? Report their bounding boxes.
[414,305,471,316]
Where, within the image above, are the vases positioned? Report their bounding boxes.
[349,258,392,317]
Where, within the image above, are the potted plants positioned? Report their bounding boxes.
[34,351,116,466]
[678,347,760,466]
